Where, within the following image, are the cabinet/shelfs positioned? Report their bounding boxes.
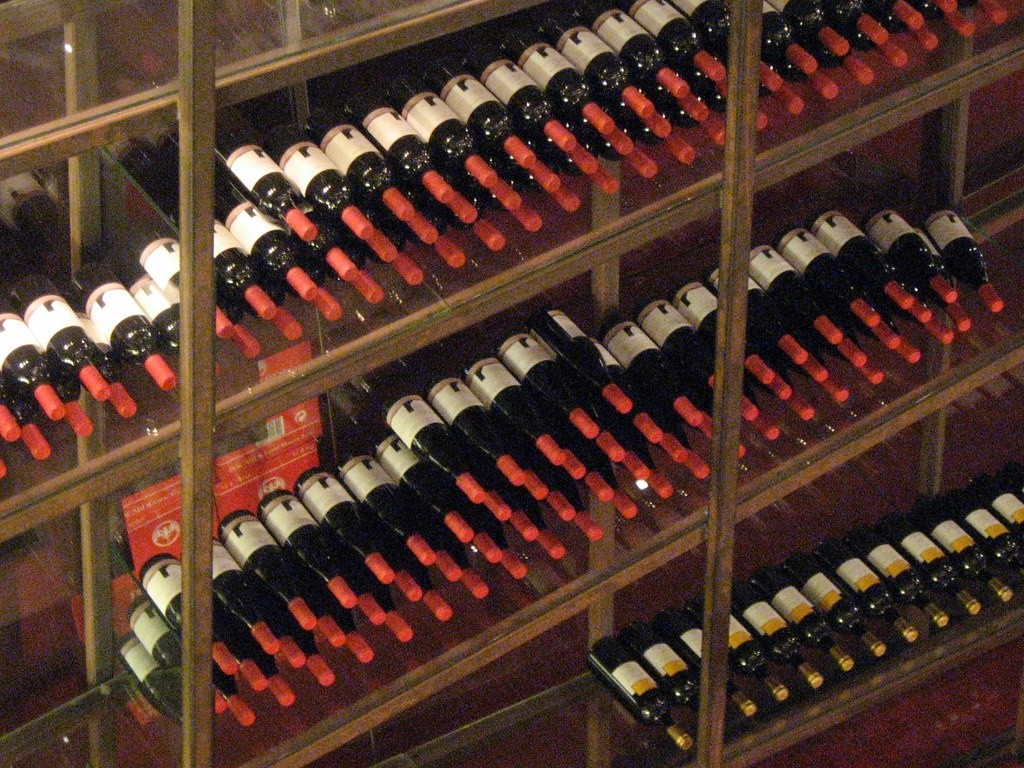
[0,0,1024,768]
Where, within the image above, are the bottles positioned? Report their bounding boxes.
[0,0,1024,768]
